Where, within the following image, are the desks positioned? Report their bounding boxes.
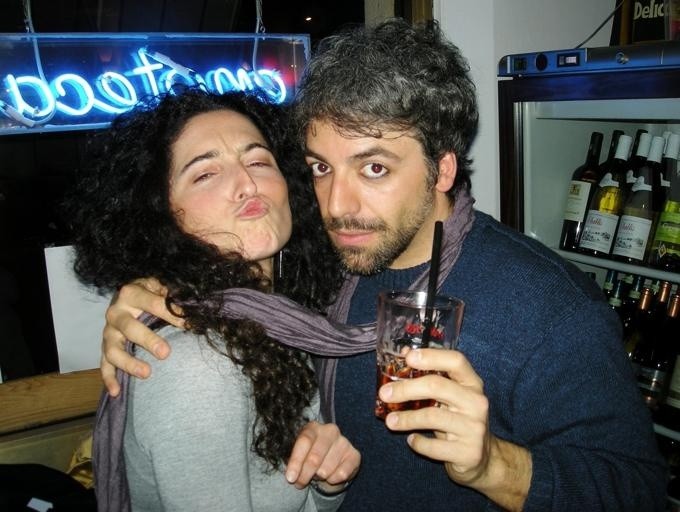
[1,367,102,474]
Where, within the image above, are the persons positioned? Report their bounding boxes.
[100,17,666,512]
[60,83,362,512]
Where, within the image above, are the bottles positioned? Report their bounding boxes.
[657,434,679,501]
[559,128,679,272]
[581,266,680,430]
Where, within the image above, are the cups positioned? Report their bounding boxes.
[375,287,464,425]
